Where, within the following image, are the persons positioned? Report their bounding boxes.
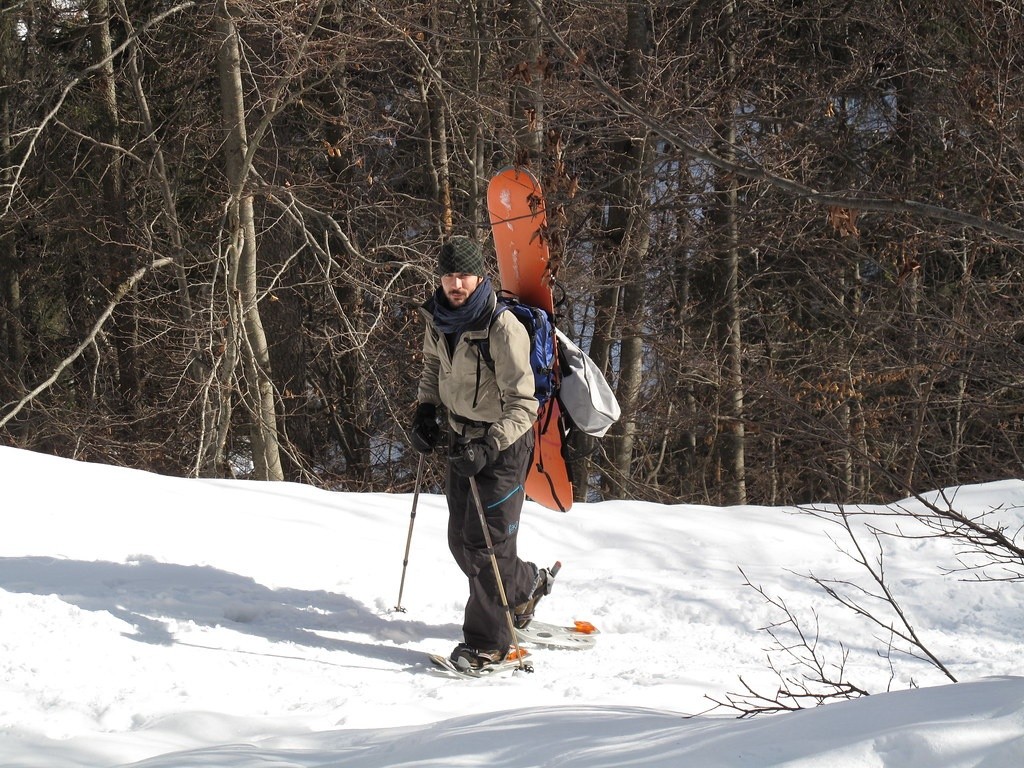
[405,231,560,679]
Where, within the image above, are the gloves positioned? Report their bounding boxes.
[450,436,499,478]
[409,403,441,456]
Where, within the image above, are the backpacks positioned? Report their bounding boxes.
[472,289,573,436]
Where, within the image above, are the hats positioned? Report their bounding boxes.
[438,238,485,279]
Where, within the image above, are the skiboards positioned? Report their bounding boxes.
[430,622,600,679]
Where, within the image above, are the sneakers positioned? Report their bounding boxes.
[448,643,509,670]
[513,568,554,629]
